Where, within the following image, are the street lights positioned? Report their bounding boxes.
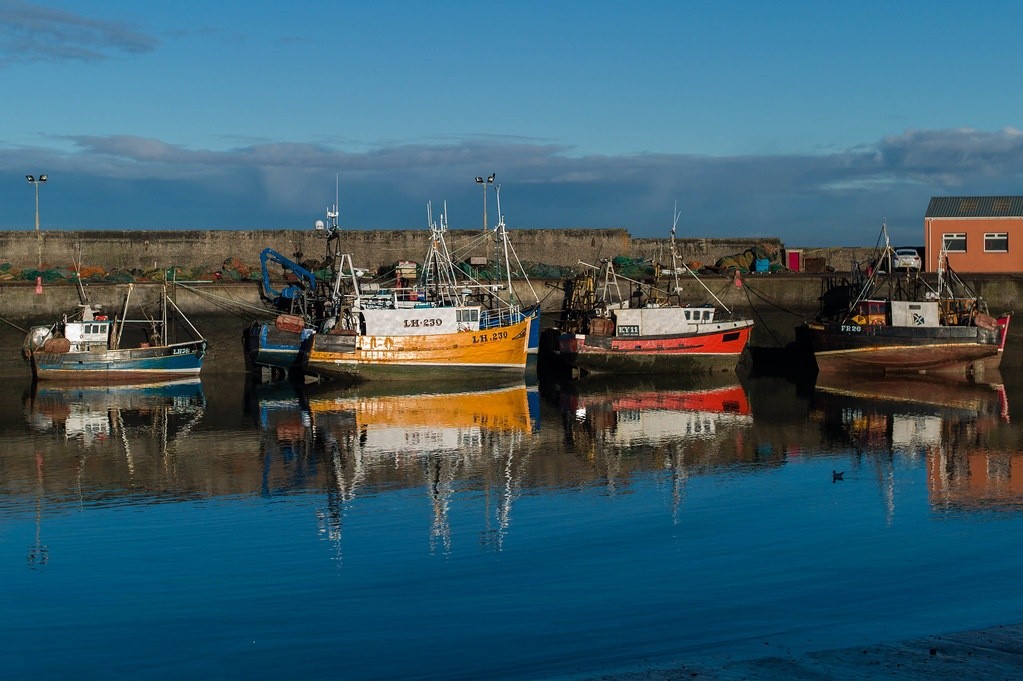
[25,171,48,270]
[472,172,497,266]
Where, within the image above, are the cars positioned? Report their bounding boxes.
[892,248,924,271]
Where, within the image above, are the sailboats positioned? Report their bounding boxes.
[255,361,543,568]
[239,227,542,369]
[566,366,755,531]
[549,225,755,364]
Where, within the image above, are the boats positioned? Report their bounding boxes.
[21,379,209,514]
[794,213,1002,371]
[814,368,1018,525]
[21,283,206,385]
